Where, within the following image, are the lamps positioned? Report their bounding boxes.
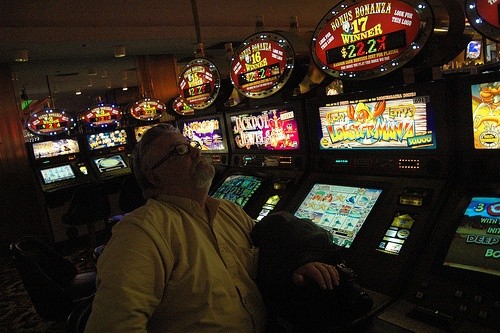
[15,50,28,62]
[75,65,128,96]
[12,72,18,81]
[192,15,298,58]
[113,47,126,58]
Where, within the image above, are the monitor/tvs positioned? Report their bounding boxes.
[32,79,500,279]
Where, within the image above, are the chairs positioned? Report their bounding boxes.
[11,172,148,333]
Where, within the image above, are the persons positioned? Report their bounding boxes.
[83,124,341,333]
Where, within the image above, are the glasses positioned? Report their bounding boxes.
[150,141,202,171]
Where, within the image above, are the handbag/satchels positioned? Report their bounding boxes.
[262,260,374,326]
